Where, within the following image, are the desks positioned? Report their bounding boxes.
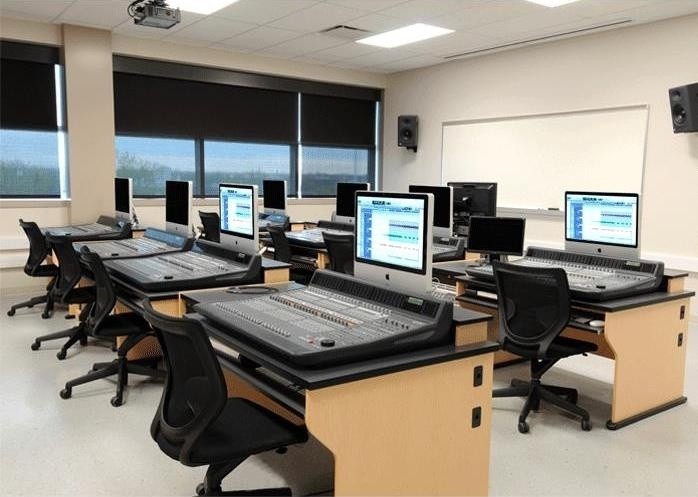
[180,272,491,494]
[429,243,464,260]
[96,238,294,364]
[64,227,197,319]
[38,215,133,293]
[283,221,353,269]
[457,245,696,431]
[256,211,289,247]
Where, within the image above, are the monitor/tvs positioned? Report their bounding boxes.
[219,184,258,253]
[165,181,192,238]
[408,185,453,237]
[353,191,433,294]
[114,177,132,221]
[262,180,288,218]
[466,215,527,266]
[448,182,498,237]
[336,182,370,228]
[565,191,642,263]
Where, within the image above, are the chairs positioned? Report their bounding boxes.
[198,210,220,242]
[6,219,62,319]
[142,298,309,497]
[31,231,117,360]
[491,260,592,433]
[266,223,314,284]
[322,231,355,277]
[60,245,167,407]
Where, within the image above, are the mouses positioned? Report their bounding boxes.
[590,319,604,327]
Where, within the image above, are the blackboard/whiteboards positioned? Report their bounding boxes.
[440,104,649,217]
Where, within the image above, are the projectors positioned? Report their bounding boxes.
[128,0,180,29]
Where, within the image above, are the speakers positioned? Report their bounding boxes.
[669,82,698,134]
[398,115,418,152]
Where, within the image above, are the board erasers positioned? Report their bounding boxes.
[548,208,559,210]
